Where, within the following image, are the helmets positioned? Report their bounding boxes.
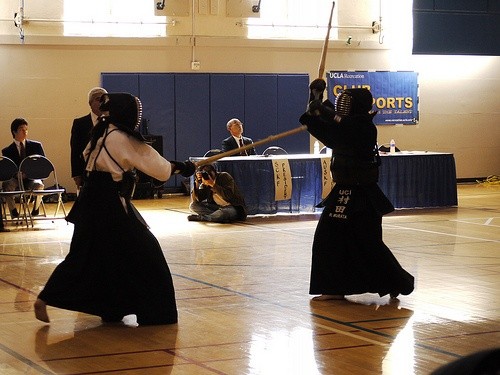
[99,92,143,128]
[334,88,372,116]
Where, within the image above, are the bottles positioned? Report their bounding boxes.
[390,139,396,153]
[314,141,319,157]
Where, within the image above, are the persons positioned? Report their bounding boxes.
[70,87,109,190]
[188,163,247,223]
[300,79,414,302]
[33,92,197,326]
[1,118,46,219]
[0,204,11,232]
[221,118,258,156]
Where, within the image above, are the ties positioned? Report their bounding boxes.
[20,142,25,160]
[239,139,245,154]
[98,117,102,121]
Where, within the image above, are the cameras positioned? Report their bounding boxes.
[201,171,210,180]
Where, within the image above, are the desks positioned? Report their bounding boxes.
[189,151,458,214]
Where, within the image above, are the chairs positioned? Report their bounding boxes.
[203,149,224,157]
[378,143,400,152]
[0,154,68,231]
[262,146,289,155]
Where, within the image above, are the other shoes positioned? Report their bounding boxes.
[188,215,195,221]
[11,208,19,218]
[31,206,39,216]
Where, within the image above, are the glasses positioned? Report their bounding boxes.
[231,123,243,127]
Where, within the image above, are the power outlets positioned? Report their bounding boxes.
[191,62,200,71]
